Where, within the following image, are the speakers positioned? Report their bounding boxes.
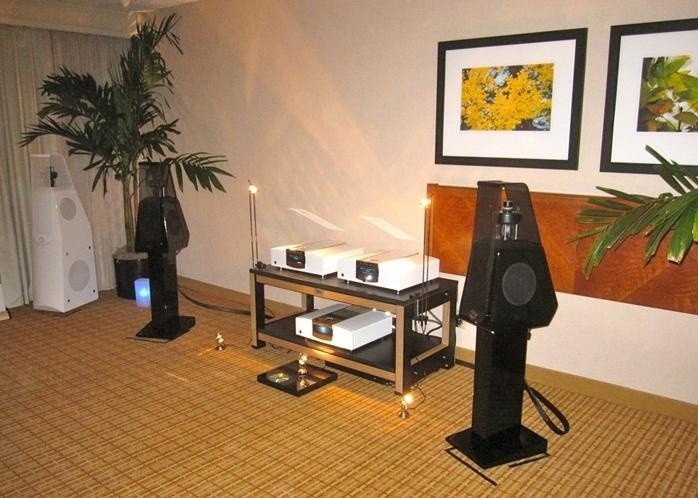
[33,188,99,311]
[459,237,556,333]
[136,200,189,254]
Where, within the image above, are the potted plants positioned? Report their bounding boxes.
[9,9,236,303]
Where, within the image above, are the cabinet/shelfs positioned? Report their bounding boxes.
[248,258,459,396]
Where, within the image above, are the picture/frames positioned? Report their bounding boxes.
[426,25,590,170]
[595,17,698,179]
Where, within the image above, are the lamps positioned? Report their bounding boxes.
[210,331,237,356]
[295,345,313,377]
[391,377,431,420]
[132,274,154,310]
